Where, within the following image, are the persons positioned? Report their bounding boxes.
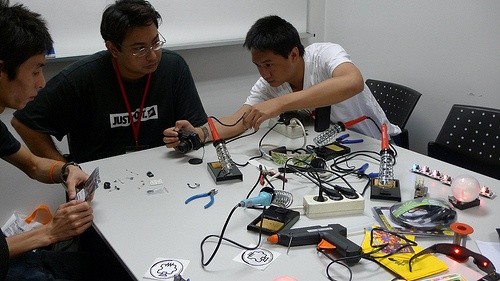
[161,15,402,149]
[1,1,105,281]
[10,0,210,255]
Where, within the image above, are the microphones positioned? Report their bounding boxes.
[238,186,276,209]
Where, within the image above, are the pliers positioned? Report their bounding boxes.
[184,188,218,209]
[260,170,287,185]
[336,133,364,144]
[358,163,381,178]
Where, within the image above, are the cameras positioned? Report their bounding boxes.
[174,129,202,153]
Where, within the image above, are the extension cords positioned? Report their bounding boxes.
[302,192,365,218]
[269,118,304,139]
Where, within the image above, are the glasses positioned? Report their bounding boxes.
[117,29,166,57]
[409,242,496,281]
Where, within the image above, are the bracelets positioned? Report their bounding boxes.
[61,161,83,185]
[49,160,61,183]
